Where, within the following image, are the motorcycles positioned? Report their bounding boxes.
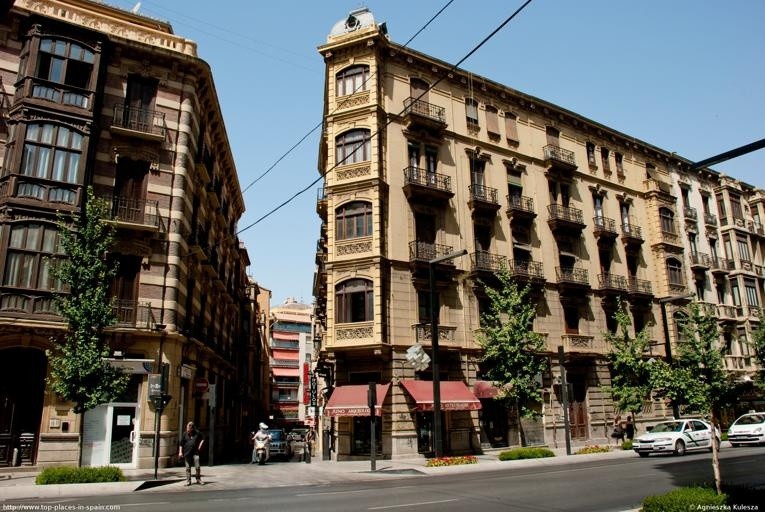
[249,430,274,464]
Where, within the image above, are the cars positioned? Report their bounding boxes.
[727,411,764,448]
[632,416,724,456]
[257,428,310,462]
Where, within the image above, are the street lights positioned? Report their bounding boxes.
[147,328,169,478]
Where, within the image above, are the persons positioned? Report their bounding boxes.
[178,421,205,485]
[286,426,316,462]
[250,422,273,464]
[613,414,625,445]
[625,416,637,440]
